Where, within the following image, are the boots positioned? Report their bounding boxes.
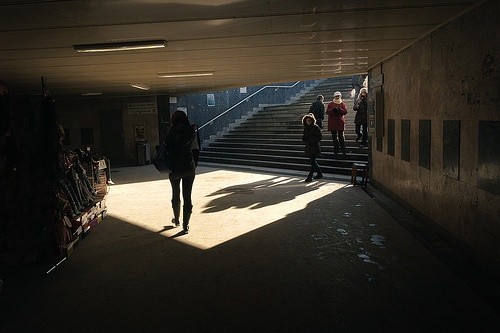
[171,199,181,226]
[182,204,192,233]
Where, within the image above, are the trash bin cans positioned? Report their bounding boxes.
[136,142,151,166]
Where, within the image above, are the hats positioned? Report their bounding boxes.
[333,91,341,96]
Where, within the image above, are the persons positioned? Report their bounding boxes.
[353,89,368,148]
[308,94,325,128]
[351,74,368,104]
[166,110,196,234]
[301,113,323,182]
[326,91,347,157]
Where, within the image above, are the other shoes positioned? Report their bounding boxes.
[107,179,115,185]
[313,173,322,179]
[305,177,312,182]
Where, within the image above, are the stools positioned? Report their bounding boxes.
[351,164,369,188]
